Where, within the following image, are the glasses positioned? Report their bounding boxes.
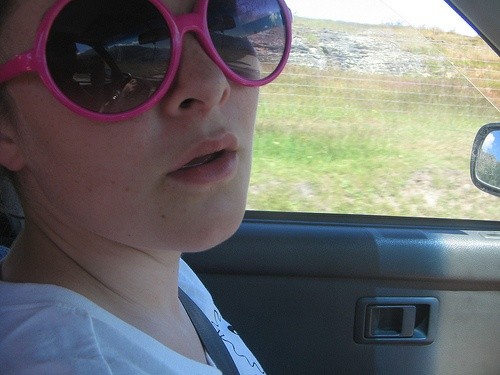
[1,0,293,120]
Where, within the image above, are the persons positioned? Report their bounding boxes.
[0,1,294,375]
